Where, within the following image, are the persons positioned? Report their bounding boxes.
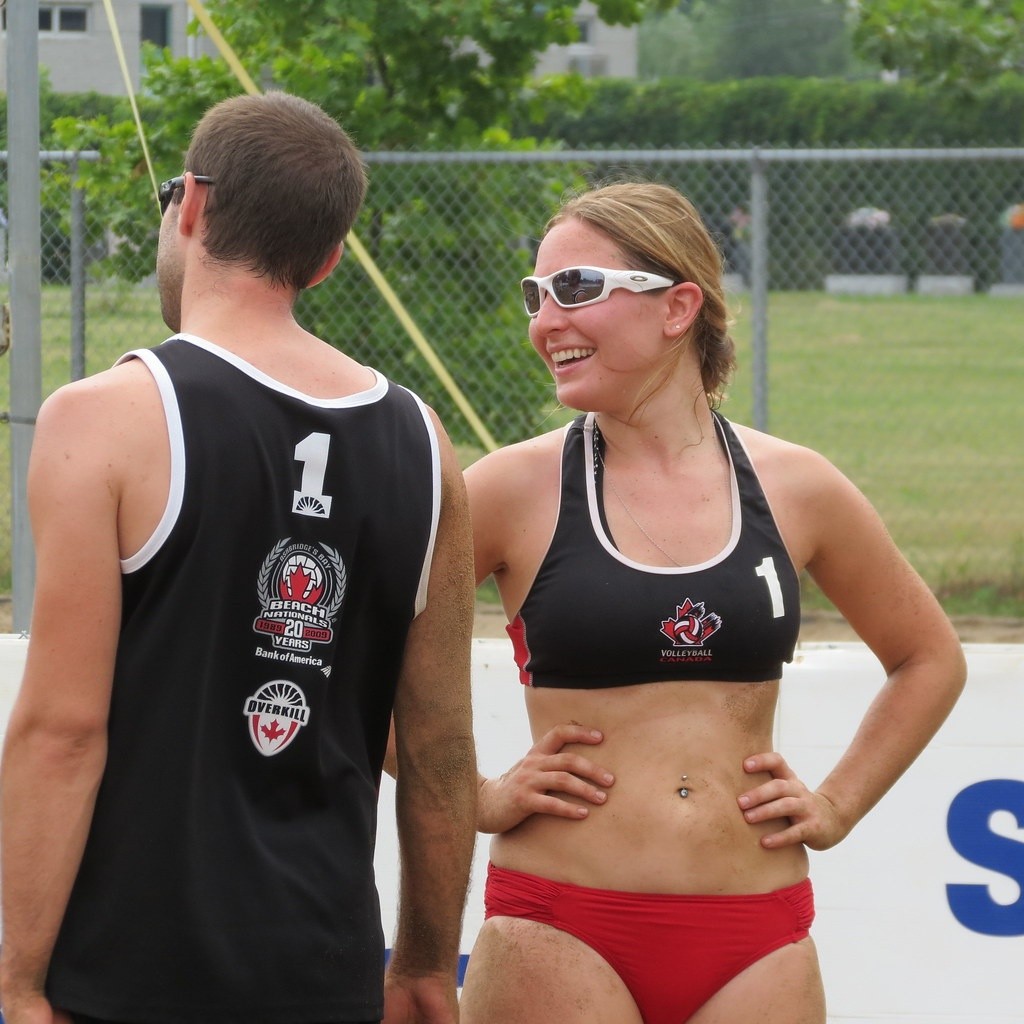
[0,88,477,1023]
[383,181,968,1024]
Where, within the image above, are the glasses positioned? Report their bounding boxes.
[159,175,214,217]
[521,265,681,317]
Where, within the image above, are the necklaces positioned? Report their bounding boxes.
[588,430,683,571]
